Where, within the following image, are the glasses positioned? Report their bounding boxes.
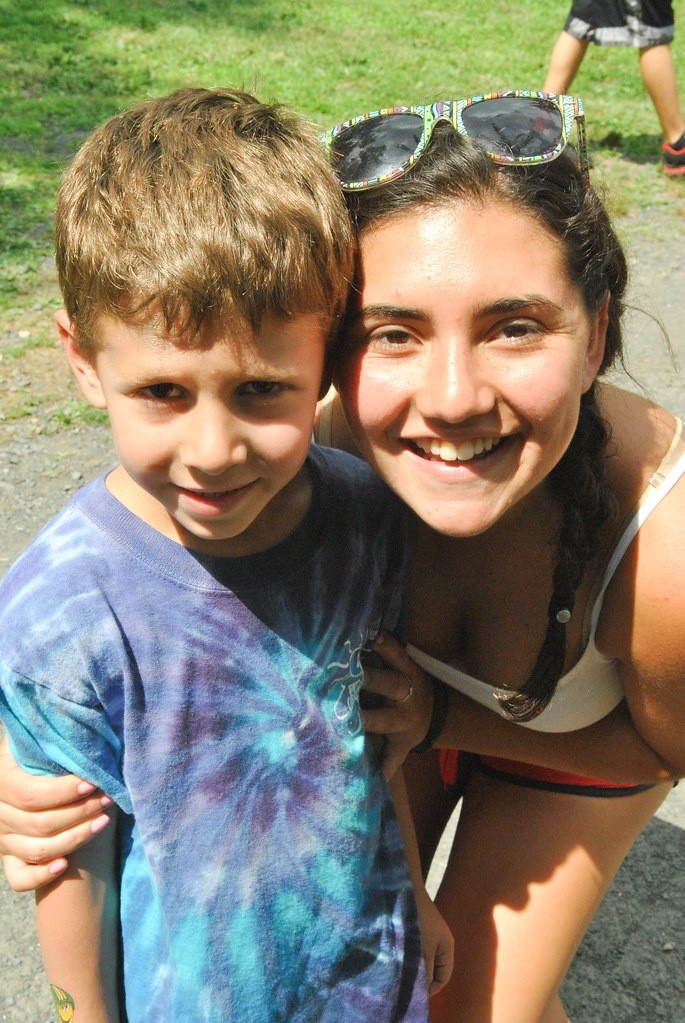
[323,92,589,193]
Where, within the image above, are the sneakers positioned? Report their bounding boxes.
[661,133,683,175]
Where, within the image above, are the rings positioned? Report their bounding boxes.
[395,674,414,703]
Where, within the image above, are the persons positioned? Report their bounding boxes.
[538,2,684,180]
[0,110,684,1020]
[1,87,460,1022]
